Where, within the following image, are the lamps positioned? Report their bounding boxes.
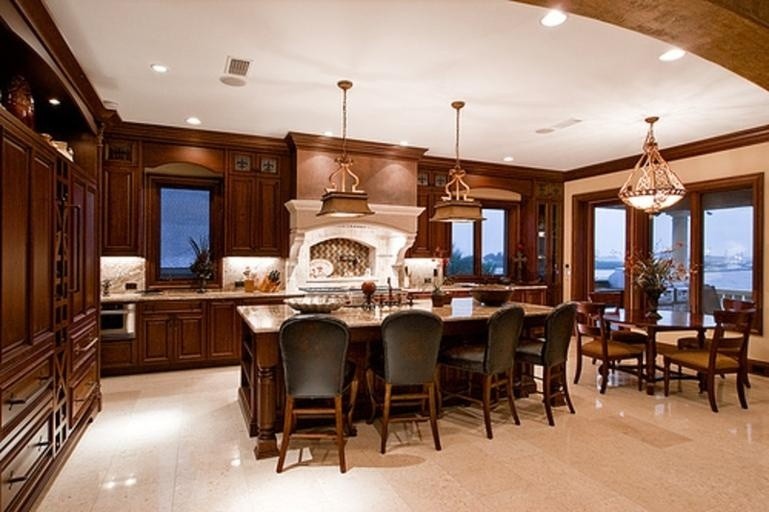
[317,79,373,218]
[428,98,485,225]
[619,112,685,213]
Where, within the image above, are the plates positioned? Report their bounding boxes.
[309,259,335,279]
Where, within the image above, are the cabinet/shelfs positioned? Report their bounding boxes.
[209,298,243,368]
[1,23,106,511]
[102,126,143,256]
[223,145,287,256]
[137,299,208,371]
[101,337,136,373]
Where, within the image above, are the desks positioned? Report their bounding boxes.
[232,296,557,461]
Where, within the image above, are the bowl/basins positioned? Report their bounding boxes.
[468,289,514,307]
[281,294,349,315]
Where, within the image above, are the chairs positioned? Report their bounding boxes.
[278,308,446,471]
[442,299,576,435]
[578,288,757,415]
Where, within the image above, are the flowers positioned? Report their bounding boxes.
[622,241,701,289]
